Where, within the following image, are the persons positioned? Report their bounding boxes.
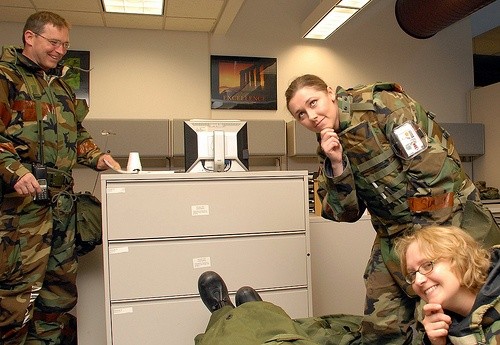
[285,73,481,315]
[0,11,121,345]
[393,225,500,345]
[194,271,320,345]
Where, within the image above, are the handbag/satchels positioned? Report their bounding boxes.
[75,191,102,257]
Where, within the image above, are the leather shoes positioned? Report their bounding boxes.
[197,271,235,314]
[235,286,263,307]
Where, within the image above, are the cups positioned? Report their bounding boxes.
[127,152,142,173]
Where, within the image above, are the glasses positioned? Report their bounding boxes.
[404,256,441,285]
[32,31,72,50]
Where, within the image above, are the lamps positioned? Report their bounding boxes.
[301,0,373,40]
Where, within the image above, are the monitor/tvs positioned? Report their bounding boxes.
[183,121,249,174]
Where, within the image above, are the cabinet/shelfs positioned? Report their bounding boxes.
[101,171,312,345]
